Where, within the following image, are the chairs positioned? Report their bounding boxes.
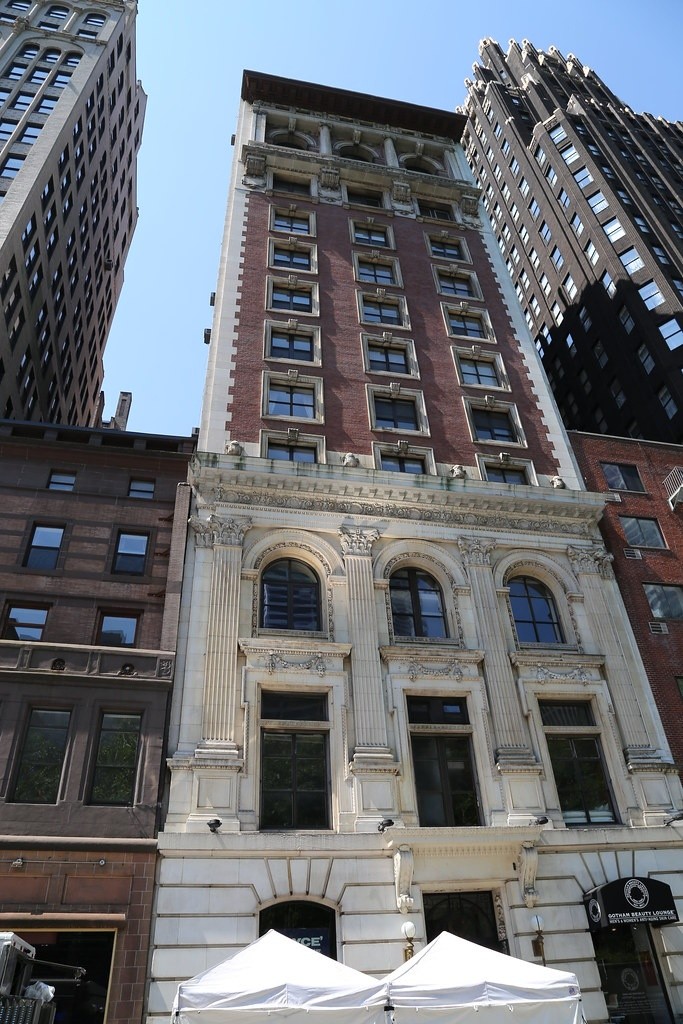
[606,994,618,1009]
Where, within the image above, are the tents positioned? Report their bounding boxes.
[167,928,384,1024]
[358,929,587,1024]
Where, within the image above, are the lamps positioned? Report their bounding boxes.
[401,921,416,962]
[532,916,547,968]
[378,819,395,833]
[666,813,683,826]
[208,819,222,832]
[531,817,548,826]
